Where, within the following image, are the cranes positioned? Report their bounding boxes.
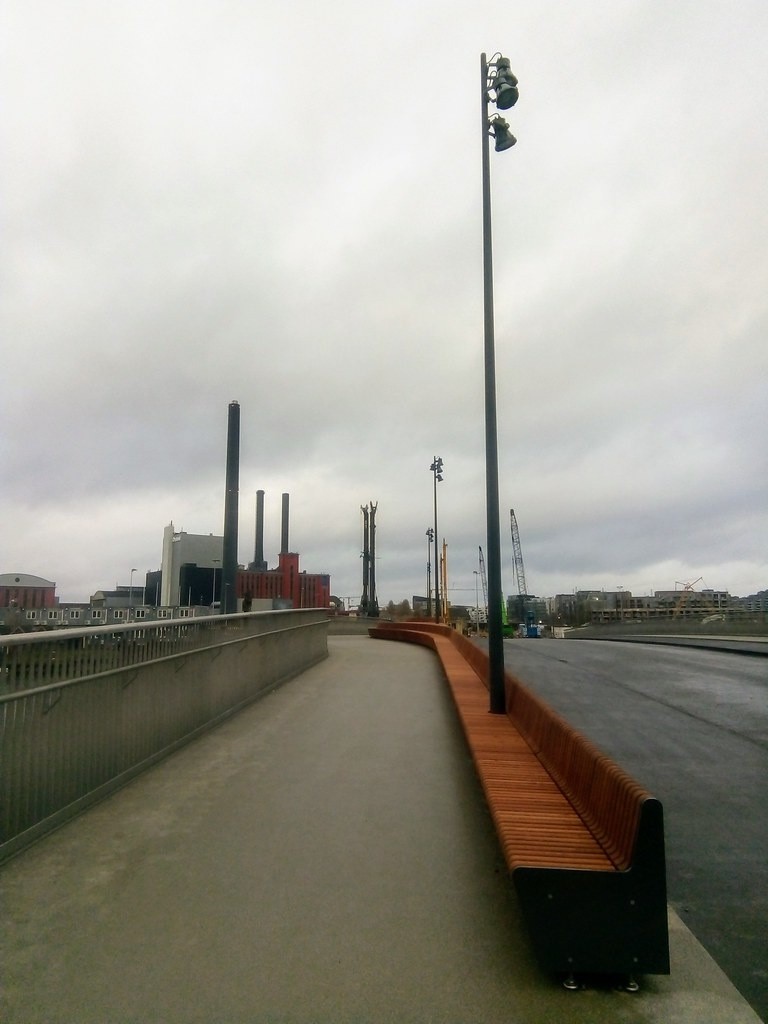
[510,509,527,595]
[671,577,715,620]
[478,546,488,607]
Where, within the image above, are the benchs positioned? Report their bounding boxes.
[368,622,670,993]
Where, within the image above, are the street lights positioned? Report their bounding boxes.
[212,560,221,609]
[430,456,443,624]
[425,528,434,617]
[129,568,137,606]
[478,52,517,712]
